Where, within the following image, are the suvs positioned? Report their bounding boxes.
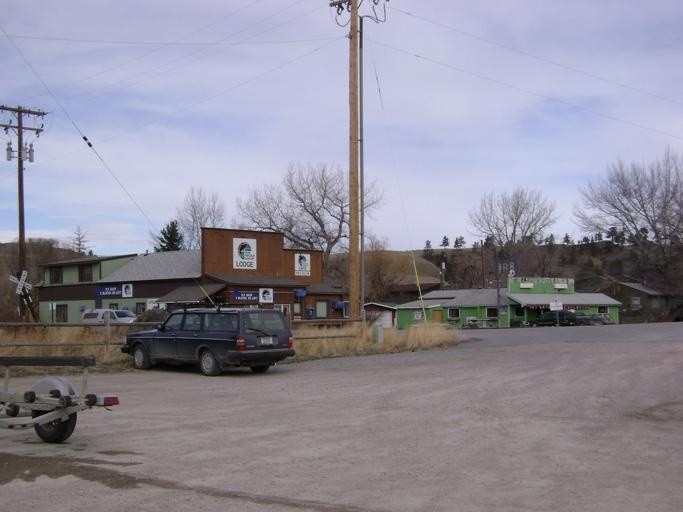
[529,310,575,327]
[123,305,295,373]
[79,308,136,325]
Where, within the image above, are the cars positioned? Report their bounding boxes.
[573,311,614,326]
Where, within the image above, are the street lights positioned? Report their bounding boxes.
[438,261,446,288]
